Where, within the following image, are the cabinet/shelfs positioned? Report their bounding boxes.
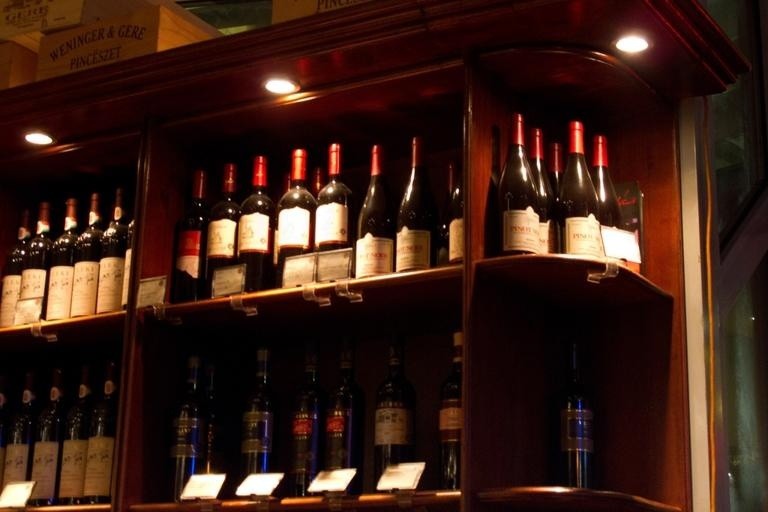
[0,1,693,512]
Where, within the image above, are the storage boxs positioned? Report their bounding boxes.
[0,30,44,90]
[272,1,370,24]
[36,2,227,80]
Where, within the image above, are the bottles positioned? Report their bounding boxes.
[355,145,394,281]
[394,137,436,271]
[168,169,206,304]
[285,357,320,498]
[323,350,355,498]
[494,110,623,260]
[234,156,274,293]
[435,332,462,492]
[169,352,216,503]
[372,346,413,494]
[206,163,239,299]
[272,149,315,288]
[0,358,118,507]
[544,340,595,490]
[312,142,352,249]
[0,187,135,330]
[236,349,272,482]
[437,164,465,268]
